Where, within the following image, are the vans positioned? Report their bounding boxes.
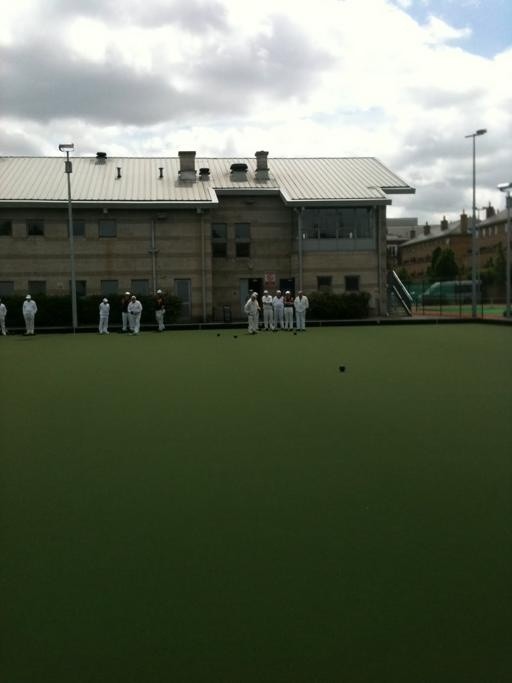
[420,280,485,303]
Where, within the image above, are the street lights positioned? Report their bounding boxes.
[59,142,76,328]
[464,127,488,316]
[497,182,512,318]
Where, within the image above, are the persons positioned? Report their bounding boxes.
[99,298,110,335]
[283,290,294,331]
[23,295,38,335]
[0,299,8,336]
[244,289,262,335]
[153,290,165,331]
[127,296,143,336]
[262,290,275,330]
[121,292,132,332]
[272,290,285,331]
[294,291,309,331]
